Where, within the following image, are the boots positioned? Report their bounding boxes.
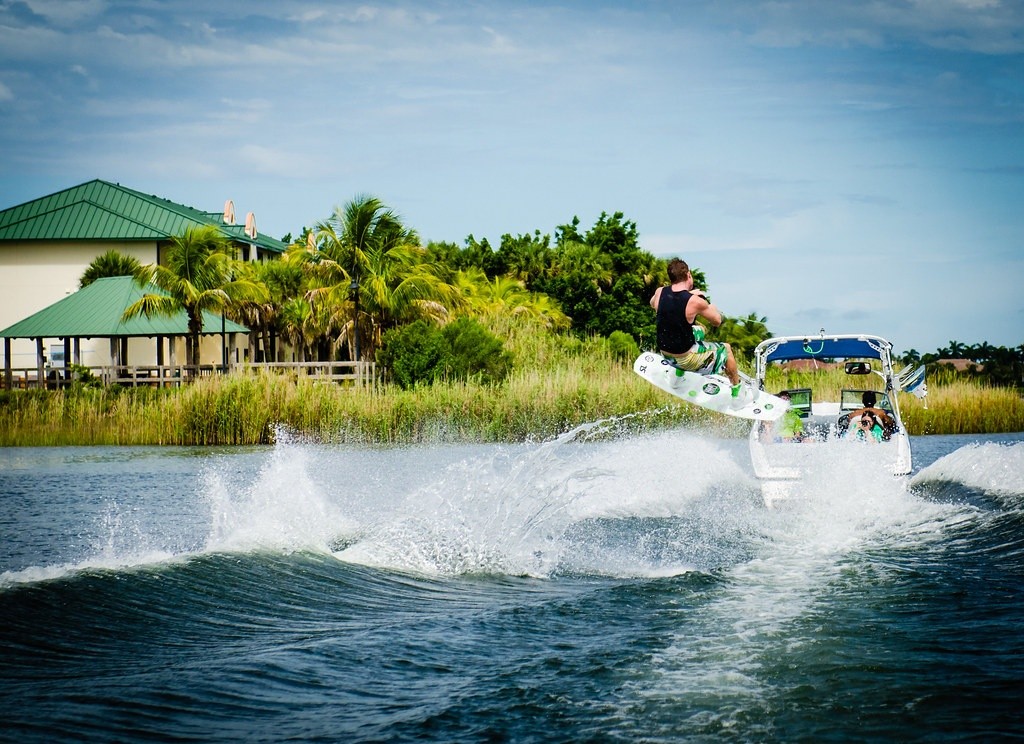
[730,379,759,411]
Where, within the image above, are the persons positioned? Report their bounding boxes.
[650,257,759,410]
[844,391,896,444]
[763,391,814,443]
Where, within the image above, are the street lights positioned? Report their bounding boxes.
[345,265,361,363]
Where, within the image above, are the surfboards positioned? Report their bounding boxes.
[632,350,791,424]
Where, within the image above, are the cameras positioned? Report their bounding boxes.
[861,420,870,427]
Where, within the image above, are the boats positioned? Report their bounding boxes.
[750,328,913,513]
